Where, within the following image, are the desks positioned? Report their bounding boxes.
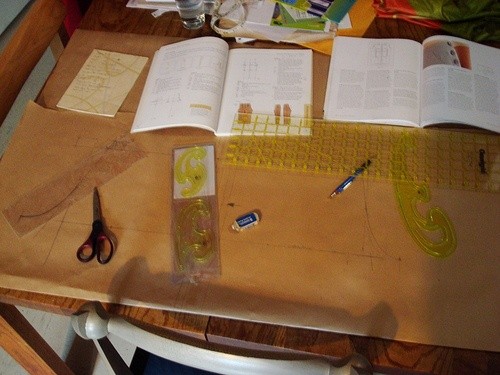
[0,0,500,375]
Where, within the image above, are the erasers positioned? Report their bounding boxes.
[235,212,259,232]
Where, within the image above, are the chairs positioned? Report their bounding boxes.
[71,299,374,375]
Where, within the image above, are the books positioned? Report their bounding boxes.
[323,35,500,133]
[131,37,314,138]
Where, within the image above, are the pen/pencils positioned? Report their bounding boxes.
[331,159,373,197]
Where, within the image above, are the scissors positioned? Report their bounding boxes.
[76,186,114,265]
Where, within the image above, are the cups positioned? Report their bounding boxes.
[175,0,221,30]
